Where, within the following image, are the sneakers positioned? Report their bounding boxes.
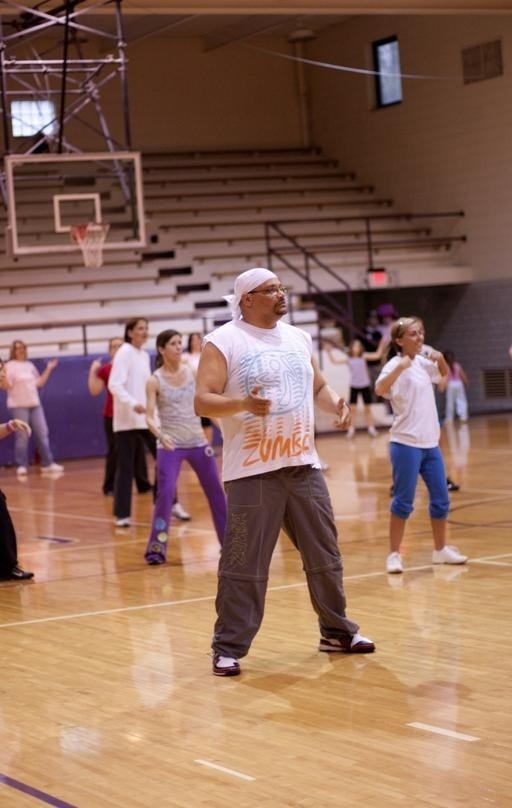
[386,553,401,573]
[172,502,190,520]
[214,655,240,676]
[319,633,375,652]
[6,567,34,581]
[433,547,467,564]
[147,554,164,565]
[446,479,459,490]
[346,425,377,437]
[16,462,63,474]
[116,517,129,527]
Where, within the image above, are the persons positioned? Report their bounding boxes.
[1,487,34,583]
[2,339,66,476]
[324,320,467,435]
[372,316,469,575]
[143,329,235,566]
[190,264,377,676]
[85,320,212,527]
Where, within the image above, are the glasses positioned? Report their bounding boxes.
[249,287,285,296]
